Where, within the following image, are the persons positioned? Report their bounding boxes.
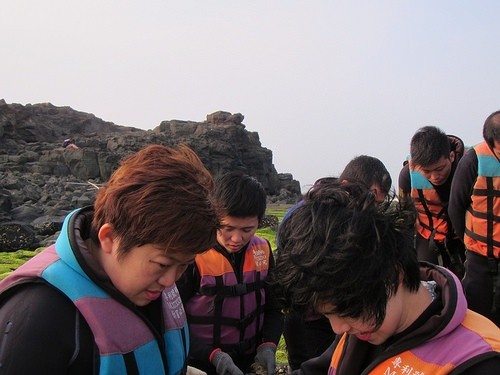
[448,112,500,327]
[277,156,393,368]
[276,183,500,375]
[0,141,219,375]
[171,172,286,375]
[399,126,468,277]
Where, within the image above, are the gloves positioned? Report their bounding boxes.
[254,342,277,375]
[209,348,244,375]
